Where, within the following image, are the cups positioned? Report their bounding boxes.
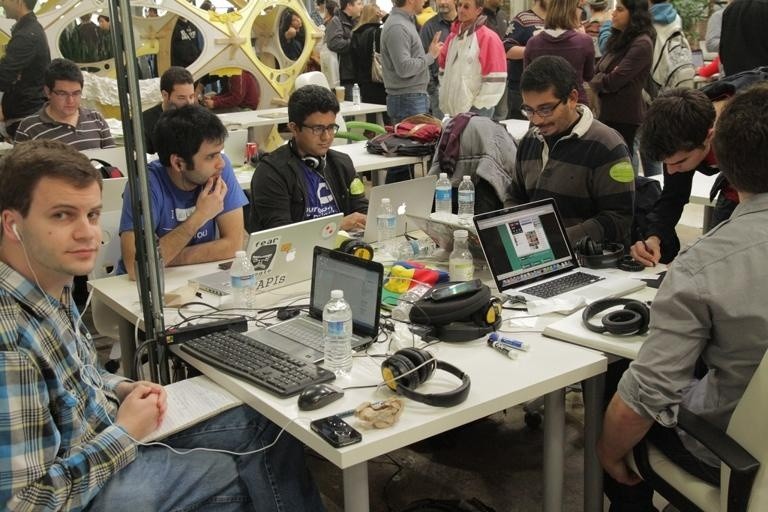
[335,86,345,103]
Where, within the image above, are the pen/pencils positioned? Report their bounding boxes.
[636,227,656,267]
[336,400,379,417]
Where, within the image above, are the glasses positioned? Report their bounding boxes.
[50,88,83,98]
[302,123,339,136]
[519,100,562,117]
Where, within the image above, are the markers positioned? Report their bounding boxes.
[487,333,530,360]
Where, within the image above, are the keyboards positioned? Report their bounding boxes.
[178,327,336,398]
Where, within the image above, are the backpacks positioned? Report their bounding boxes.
[644,26,695,105]
[73,158,127,304]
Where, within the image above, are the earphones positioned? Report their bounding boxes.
[7,221,21,242]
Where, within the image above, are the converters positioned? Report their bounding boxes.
[220,292,239,310]
[159,315,249,347]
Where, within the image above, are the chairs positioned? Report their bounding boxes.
[88,178,139,343]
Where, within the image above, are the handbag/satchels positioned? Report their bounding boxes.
[367,112,442,158]
[372,51,386,83]
[320,46,340,89]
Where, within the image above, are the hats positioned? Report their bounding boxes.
[382,260,449,305]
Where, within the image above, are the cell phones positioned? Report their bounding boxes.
[310,414,364,449]
[429,278,484,301]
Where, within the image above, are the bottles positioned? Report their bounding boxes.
[322,288,353,379]
[230,249,257,313]
[352,83,361,110]
[457,175,476,226]
[376,198,397,258]
[392,283,434,322]
[388,238,437,260]
[435,172,453,220]
[448,228,475,283]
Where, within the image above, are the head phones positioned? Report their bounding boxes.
[329,237,375,261]
[289,137,330,170]
[380,346,472,406]
[573,233,628,271]
[581,296,651,339]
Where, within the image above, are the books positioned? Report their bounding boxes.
[132,374,246,445]
[540,286,658,361]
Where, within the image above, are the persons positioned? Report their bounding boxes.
[504,55,636,253]
[0,139,329,511]
[116,103,250,282]
[247,85,370,235]
[0,0,767,154]
[629,67,768,267]
[596,84,768,511]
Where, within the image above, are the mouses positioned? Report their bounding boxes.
[298,383,345,412]
[276,306,301,320]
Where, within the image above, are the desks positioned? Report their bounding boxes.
[90,261,609,511]
[240,143,428,192]
[216,101,386,158]
[384,215,657,512]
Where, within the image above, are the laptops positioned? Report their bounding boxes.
[404,212,488,267]
[244,244,383,364]
[473,197,647,315]
[187,211,345,296]
[349,174,438,243]
